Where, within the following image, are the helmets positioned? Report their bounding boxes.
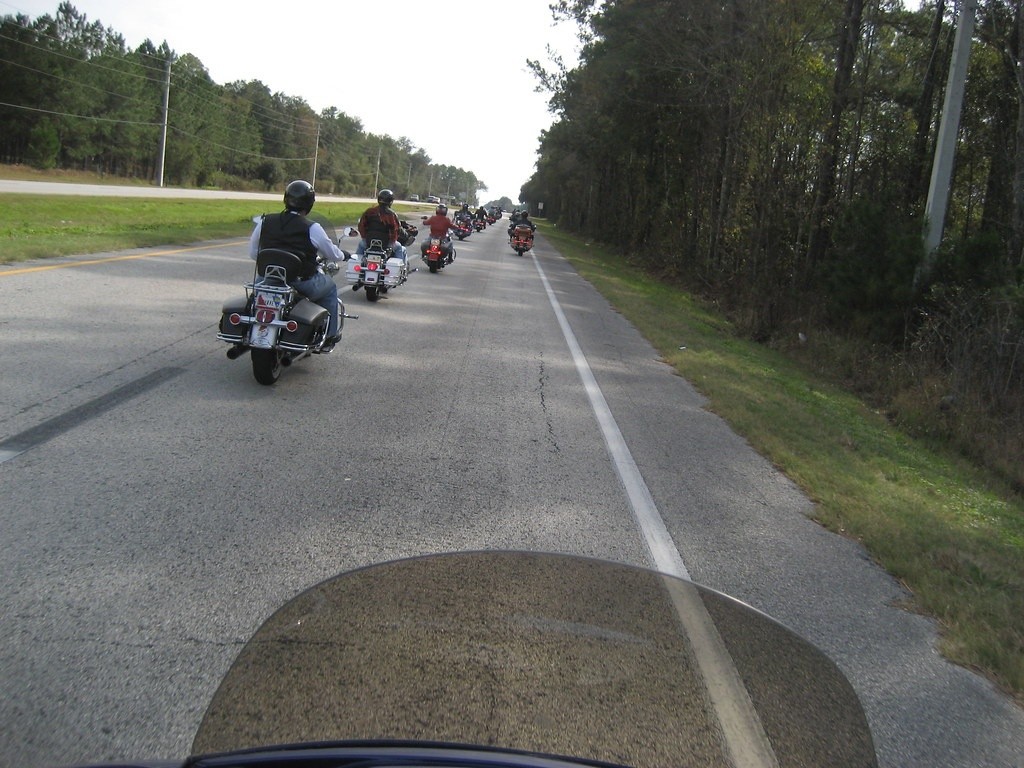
[378,189,394,207]
[522,210,528,218]
[285,180,315,215]
[436,204,448,216]
[464,204,468,208]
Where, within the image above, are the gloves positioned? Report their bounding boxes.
[342,249,350,261]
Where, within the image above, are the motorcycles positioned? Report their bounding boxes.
[344,219,419,302]
[410,192,538,257]
[419,213,461,274]
[215,209,360,385]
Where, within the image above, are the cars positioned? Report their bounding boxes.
[427,196,434,202]
[433,197,441,203]
[410,194,420,202]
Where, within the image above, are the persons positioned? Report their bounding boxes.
[352,190,535,291]
[249,181,344,344]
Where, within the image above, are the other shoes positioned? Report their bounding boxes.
[326,333,342,345]
